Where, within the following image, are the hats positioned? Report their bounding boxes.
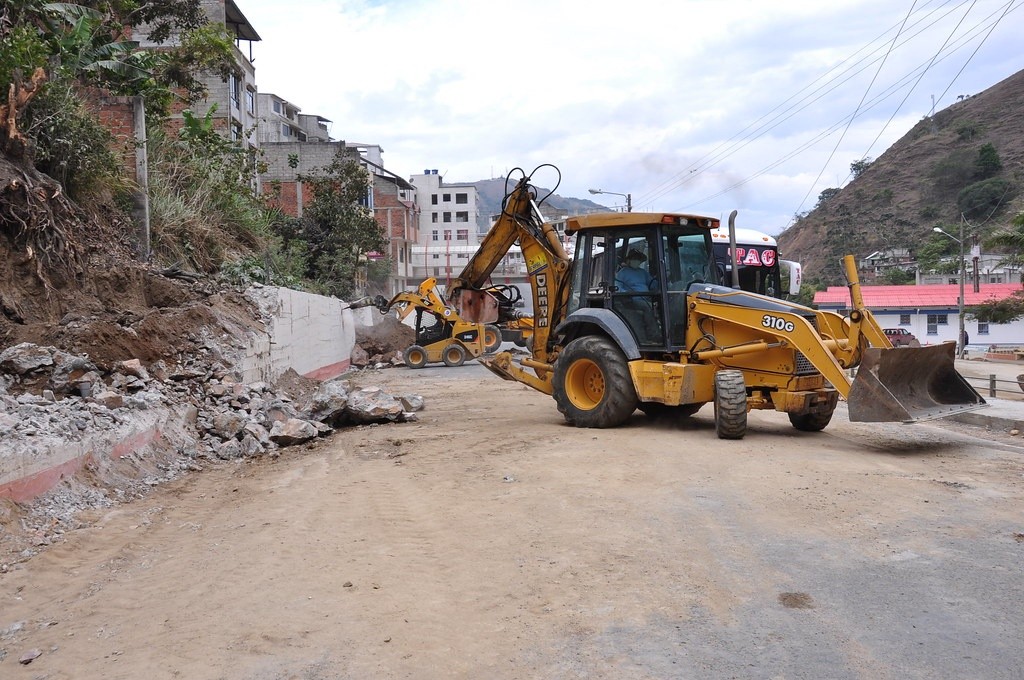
[625,248,647,264]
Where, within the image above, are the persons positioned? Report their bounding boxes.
[614,248,665,343]
[965,331,968,354]
[889,330,896,334]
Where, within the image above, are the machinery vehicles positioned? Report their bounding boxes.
[341,275,563,369]
[441,163,994,440]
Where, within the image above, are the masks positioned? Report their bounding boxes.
[629,259,640,269]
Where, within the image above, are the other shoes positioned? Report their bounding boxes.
[647,335,663,344]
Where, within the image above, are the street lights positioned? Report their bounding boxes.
[934,226,965,359]
[589,188,633,212]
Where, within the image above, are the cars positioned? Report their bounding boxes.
[881,327,916,348]
